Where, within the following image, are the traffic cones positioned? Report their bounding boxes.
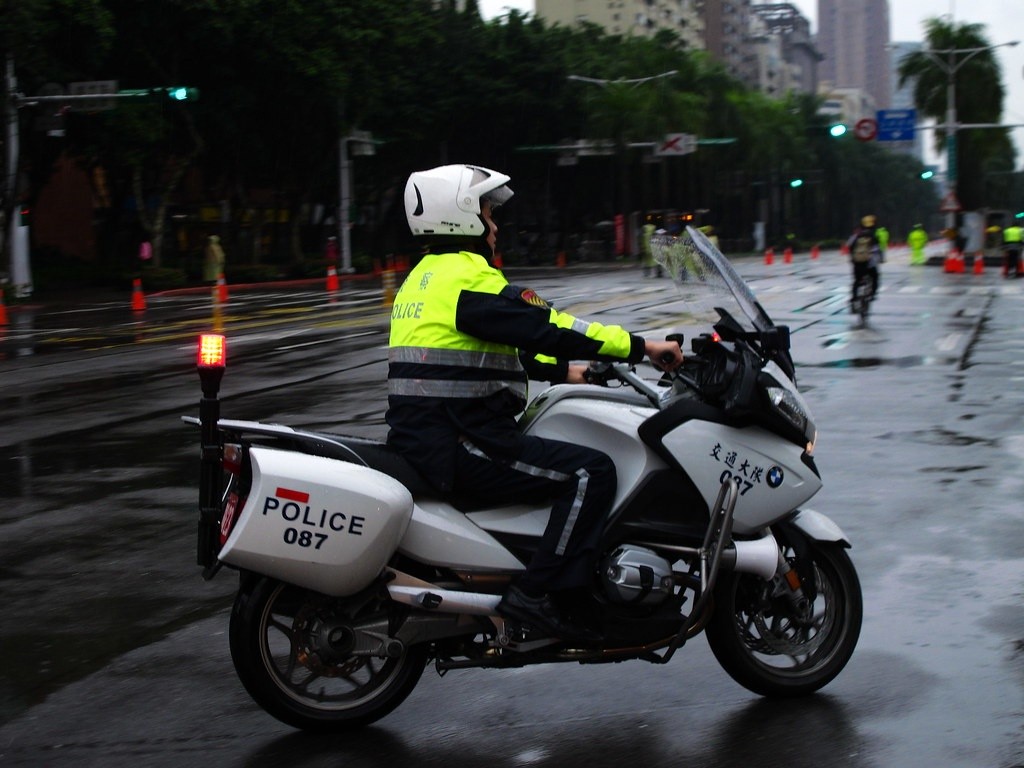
[944,247,968,273]
[127,278,148,310]
[783,246,794,263]
[0,291,10,325]
[179,221,865,733]
[215,273,230,300]
[811,244,820,258]
[326,265,339,290]
[974,251,985,274]
[764,248,774,263]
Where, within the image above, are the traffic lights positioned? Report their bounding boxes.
[784,180,803,188]
[167,87,199,101]
[916,170,934,177]
[803,126,847,137]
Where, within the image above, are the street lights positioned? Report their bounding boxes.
[884,39,1020,240]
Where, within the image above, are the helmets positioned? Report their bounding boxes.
[861,214,878,225]
[404,161,511,240]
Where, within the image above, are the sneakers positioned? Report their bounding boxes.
[493,584,605,645]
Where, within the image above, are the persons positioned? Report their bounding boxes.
[204,235,225,285]
[643,224,718,283]
[385,163,683,646]
[1000,220,1024,270]
[907,224,926,265]
[325,236,337,257]
[848,215,881,302]
[875,225,889,264]
